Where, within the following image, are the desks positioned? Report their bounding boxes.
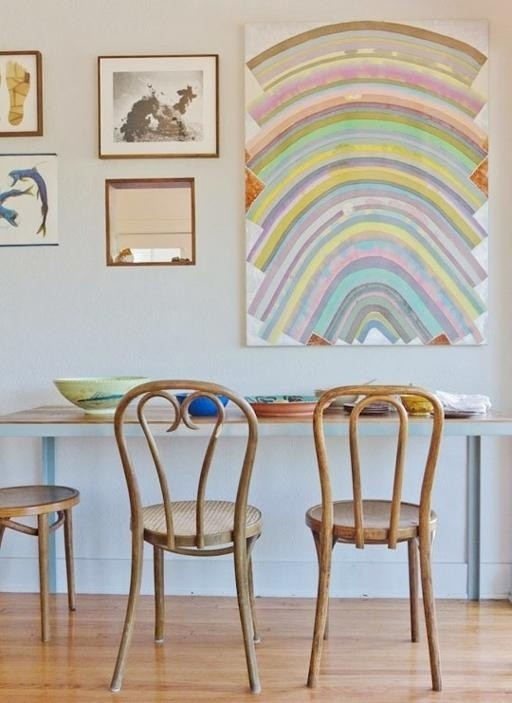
[1,396,510,605]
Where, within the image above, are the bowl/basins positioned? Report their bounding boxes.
[313,387,359,410]
[399,394,434,417]
[53,377,151,415]
[172,392,230,417]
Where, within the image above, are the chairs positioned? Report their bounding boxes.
[107,379,264,693]
[303,384,445,692]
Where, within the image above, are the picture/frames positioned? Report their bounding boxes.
[94,50,221,271]
[0,46,43,135]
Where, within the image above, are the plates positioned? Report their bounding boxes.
[342,402,393,416]
[241,395,336,417]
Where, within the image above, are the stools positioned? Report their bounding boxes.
[2,485,81,644]
